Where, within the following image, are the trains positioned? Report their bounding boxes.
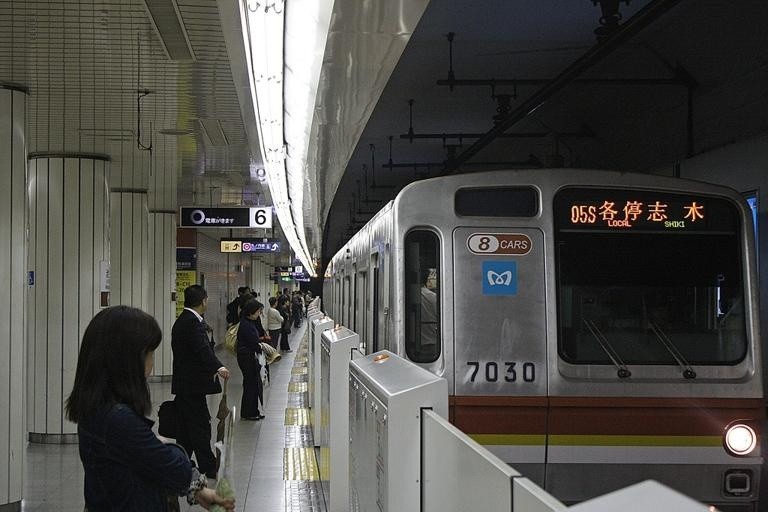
[321,170,768,510]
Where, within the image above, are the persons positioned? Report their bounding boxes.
[60,303,237,512]
[236,299,269,421]
[226,285,316,392]
[169,284,232,482]
[420,264,438,357]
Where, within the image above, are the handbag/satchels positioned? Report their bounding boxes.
[157,401,184,438]
[224,322,240,356]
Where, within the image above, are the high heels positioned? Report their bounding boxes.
[242,409,262,421]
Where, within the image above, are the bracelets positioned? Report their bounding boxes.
[187,473,209,507]
